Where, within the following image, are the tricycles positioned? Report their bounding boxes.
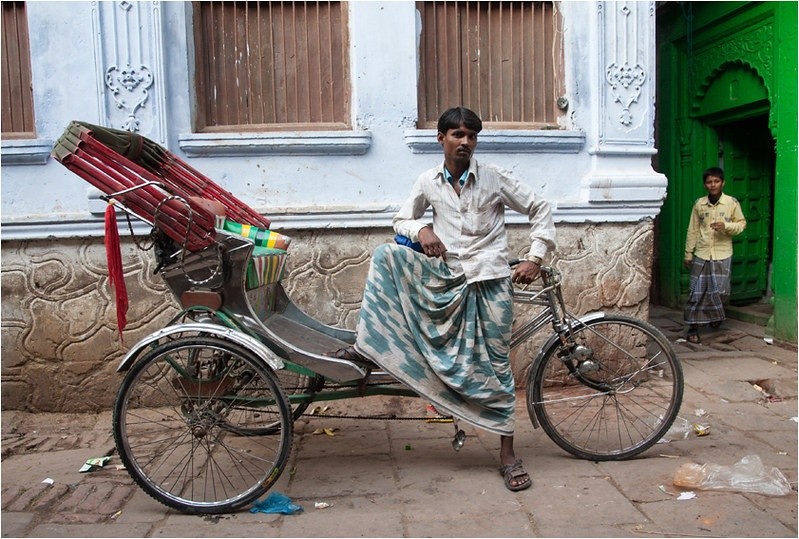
[50,119,681,515]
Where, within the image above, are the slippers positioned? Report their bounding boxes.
[499,460,532,492]
[686,328,701,343]
[710,321,720,328]
[322,345,381,369]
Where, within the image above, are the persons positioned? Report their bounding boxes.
[322,107,557,492]
[684,167,747,343]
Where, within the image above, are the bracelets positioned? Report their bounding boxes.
[526,255,544,265]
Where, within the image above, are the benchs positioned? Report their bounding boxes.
[182,194,293,292]
